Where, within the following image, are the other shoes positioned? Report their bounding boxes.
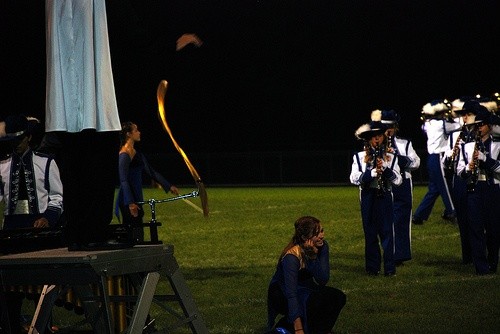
[413,215,423,224]
[444,209,456,219]
[364,270,381,276]
[474,264,489,275]
[488,259,499,273]
[384,272,395,277]
[398,257,411,261]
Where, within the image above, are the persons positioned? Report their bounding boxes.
[115,122,180,242]
[350,120,403,277]
[414,97,500,274]
[267,216,346,334]
[379,110,420,261]
[0,111,64,334]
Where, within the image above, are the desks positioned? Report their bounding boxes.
[0,243,209,334]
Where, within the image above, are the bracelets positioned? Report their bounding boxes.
[295,328,303,332]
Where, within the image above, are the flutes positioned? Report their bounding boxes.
[466,131,482,193]
[388,137,392,151]
[444,122,466,175]
[375,146,382,197]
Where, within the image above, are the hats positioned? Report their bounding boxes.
[0,115,38,142]
[423,99,450,115]
[454,100,488,115]
[355,121,387,140]
[371,109,400,124]
[451,98,465,111]
